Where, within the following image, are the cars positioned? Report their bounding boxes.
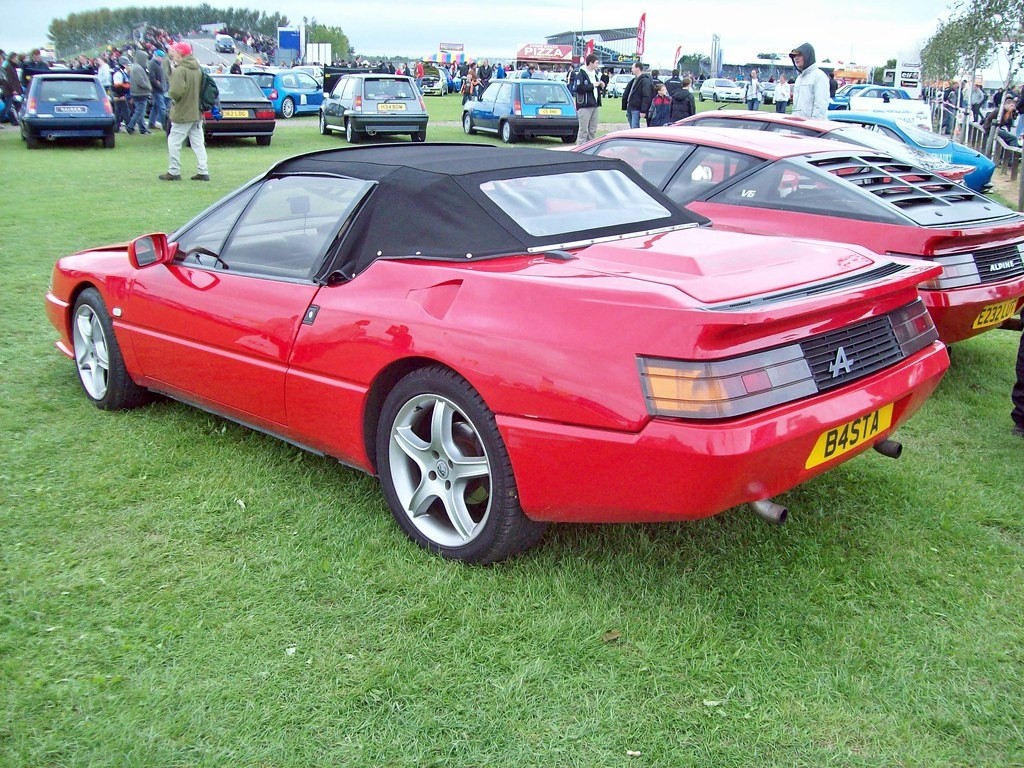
[607,74,637,98]
[209,64,381,95]
[420,64,568,97]
[12,71,118,150]
[318,73,429,144]
[163,73,276,145]
[698,78,745,105]
[734,80,912,110]
[460,78,580,145]
[243,68,325,120]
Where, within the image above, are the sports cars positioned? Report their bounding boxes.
[656,107,978,186]
[827,110,999,196]
[542,123,1024,357]
[41,141,953,570]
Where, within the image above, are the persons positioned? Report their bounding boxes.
[255,57,271,66]
[378,60,396,74]
[395,63,411,75]
[922,79,1024,167]
[331,56,361,68]
[621,42,887,129]
[278,58,298,69]
[214,25,278,56]
[186,27,208,36]
[70,25,183,136]
[216,64,223,74]
[230,59,242,74]
[158,42,211,181]
[237,50,244,64]
[416,61,425,96]
[492,60,536,79]
[555,53,626,146]
[439,59,492,109]
[0,48,50,129]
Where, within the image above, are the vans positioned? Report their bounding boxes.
[214,34,236,53]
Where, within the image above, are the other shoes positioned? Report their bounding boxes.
[116,121,161,136]
[159,172,181,180]
[191,174,210,181]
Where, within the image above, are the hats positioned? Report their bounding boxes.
[170,42,193,56]
[789,50,800,58]
[118,56,131,64]
[154,50,166,57]
[0,48,9,58]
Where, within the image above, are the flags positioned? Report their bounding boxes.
[636,13,646,56]
[584,39,593,64]
[674,47,680,68]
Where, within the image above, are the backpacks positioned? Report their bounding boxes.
[199,66,220,113]
